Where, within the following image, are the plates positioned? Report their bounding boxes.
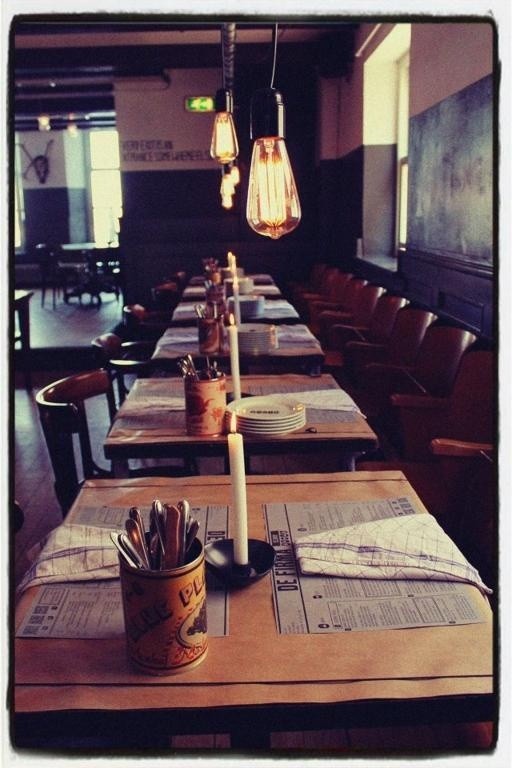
[224,325,278,354]
[224,277,253,295]
[222,266,245,281]
[229,295,264,317]
[226,396,305,434]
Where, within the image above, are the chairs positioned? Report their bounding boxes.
[13,240,204,520]
[286,240,498,550]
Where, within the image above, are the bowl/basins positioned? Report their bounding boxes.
[205,537,277,585]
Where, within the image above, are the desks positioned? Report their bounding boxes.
[13,267,497,751]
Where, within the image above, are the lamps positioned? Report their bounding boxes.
[243,20,303,238]
[206,23,240,163]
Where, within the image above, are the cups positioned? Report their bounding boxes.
[119,532,209,679]
[198,268,228,355]
[182,373,227,434]
